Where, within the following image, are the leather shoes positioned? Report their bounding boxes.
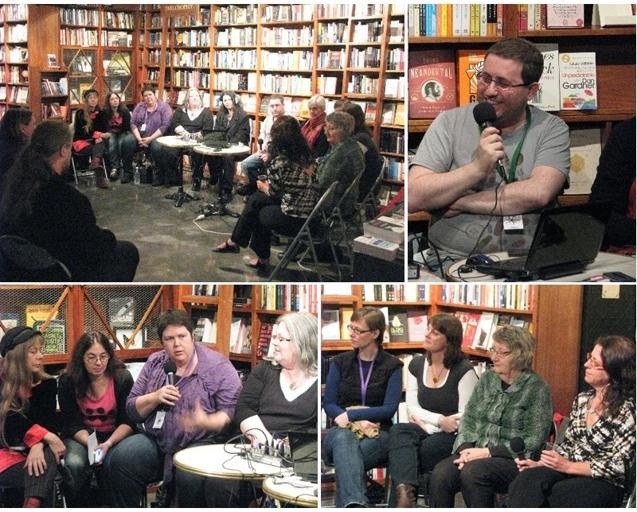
[211,240,240,252]
[243,257,272,268]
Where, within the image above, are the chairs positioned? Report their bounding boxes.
[262,151,396,281]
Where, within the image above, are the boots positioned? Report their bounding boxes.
[396,485,417,507]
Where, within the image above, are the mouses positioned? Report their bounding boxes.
[466,253,494,269]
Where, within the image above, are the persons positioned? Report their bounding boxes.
[408,36,570,281]
[585,117,636,258]
[0,84,385,283]
[1,313,317,508]
[321,308,634,507]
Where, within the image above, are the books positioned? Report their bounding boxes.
[408,3,637,196]
[0,5,404,183]
[353,215,404,263]
[0,285,318,384]
[321,284,530,384]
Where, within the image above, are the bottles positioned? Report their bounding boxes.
[133,166,140,185]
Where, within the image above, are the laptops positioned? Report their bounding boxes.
[477,198,617,280]
[287,429,318,485]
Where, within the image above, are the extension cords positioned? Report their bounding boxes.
[244,446,288,468]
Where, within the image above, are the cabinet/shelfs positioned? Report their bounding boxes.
[320,285,584,417]
[408,5,636,212]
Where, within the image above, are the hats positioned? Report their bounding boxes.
[1,325,42,357]
[84,90,98,98]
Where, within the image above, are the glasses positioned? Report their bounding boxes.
[347,325,370,334]
[476,71,530,88]
[84,354,110,363]
[488,347,511,358]
[272,335,291,342]
[586,352,602,368]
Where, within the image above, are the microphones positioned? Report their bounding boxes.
[164,360,179,402]
[509,436,529,466]
[258,139,264,151]
[471,100,512,181]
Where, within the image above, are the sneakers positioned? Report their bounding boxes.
[96,177,110,188]
[121,172,131,183]
[192,179,201,191]
[223,189,233,200]
[110,169,119,179]
